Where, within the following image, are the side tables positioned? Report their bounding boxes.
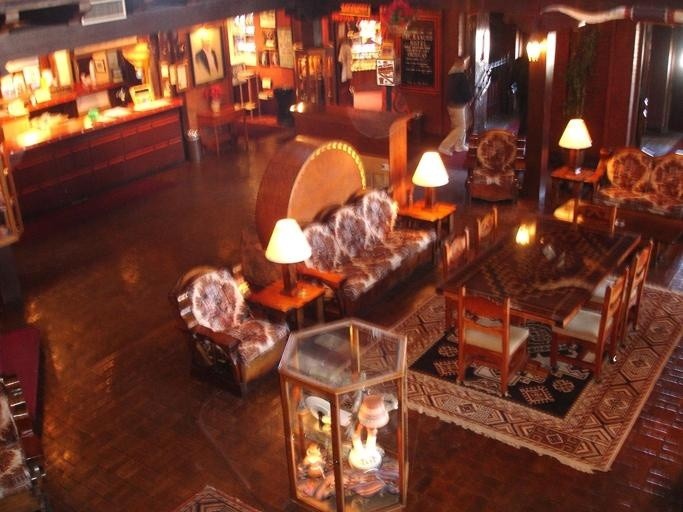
[248,279,325,331]
[399,200,456,246]
[550,167,599,223]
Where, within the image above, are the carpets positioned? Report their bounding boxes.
[360,282,683,475]
[170,483,261,512]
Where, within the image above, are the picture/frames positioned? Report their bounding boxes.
[186,25,228,89]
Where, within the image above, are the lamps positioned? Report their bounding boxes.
[558,118,593,175]
[411,150,451,211]
[265,217,313,295]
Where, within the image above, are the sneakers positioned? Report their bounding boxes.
[438,146,453,156]
[454,145,470,152]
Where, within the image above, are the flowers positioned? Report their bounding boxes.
[201,83,224,100]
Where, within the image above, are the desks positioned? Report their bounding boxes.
[197,102,250,157]
[436,216,637,371]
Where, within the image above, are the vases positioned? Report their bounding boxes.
[210,100,222,112]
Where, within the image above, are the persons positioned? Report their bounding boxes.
[195,34,222,77]
[438,55,474,157]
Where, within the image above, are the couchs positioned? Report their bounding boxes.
[1,372,60,511]
[465,133,523,208]
[168,265,291,399]
[234,66,297,105]
[297,189,438,319]
[580,147,683,244]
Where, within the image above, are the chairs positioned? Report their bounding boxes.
[592,241,653,348]
[475,206,498,255]
[456,286,530,398]
[442,226,471,337]
[572,201,617,237]
[551,268,629,384]
